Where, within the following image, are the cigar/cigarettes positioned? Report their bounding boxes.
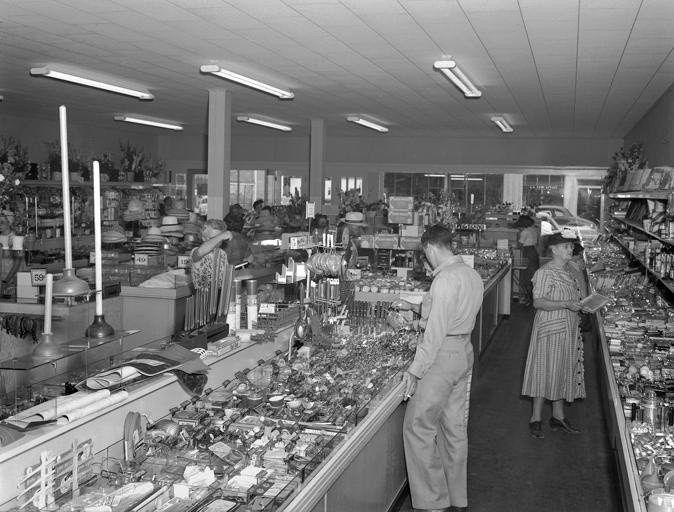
[407,394,413,400]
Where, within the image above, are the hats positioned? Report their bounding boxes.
[166,199,189,216]
[252,210,280,240]
[102,224,126,244]
[515,215,535,228]
[123,199,146,221]
[544,231,579,247]
[134,216,201,255]
[339,211,367,228]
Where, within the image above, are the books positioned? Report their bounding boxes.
[580,294,611,315]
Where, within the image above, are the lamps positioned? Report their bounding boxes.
[197,63,294,104]
[232,112,292,134]
[111,110,183,134]
[339,111,394,134]
[24,64,155,103]
[428,59,483,99]
[488,114,515,134]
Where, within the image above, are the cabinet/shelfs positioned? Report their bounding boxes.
[574,168,673,512]
[0,168,538,512]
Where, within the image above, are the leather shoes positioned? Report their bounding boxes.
[529,418,545,439]
[549,416,582,434]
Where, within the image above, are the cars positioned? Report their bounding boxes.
[535,204,598,240]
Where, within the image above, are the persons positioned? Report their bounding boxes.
[401,226,485,512]
[522,233,590,441]
[0,210,28,284]
